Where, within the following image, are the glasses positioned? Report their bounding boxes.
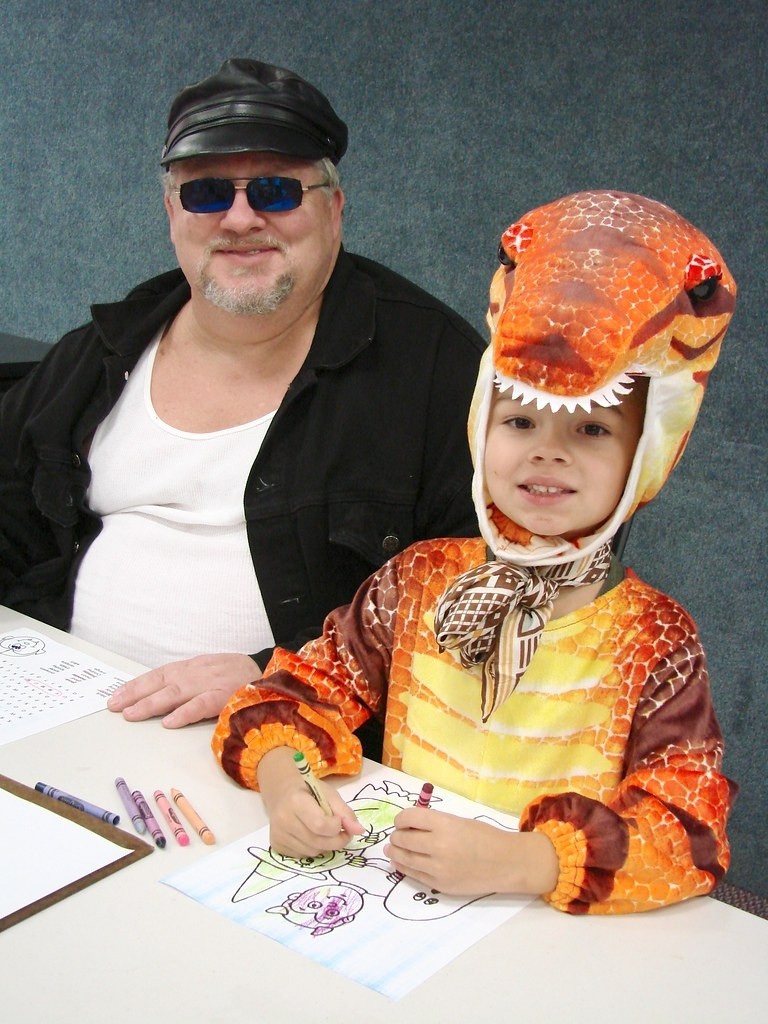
[169,175,329,214]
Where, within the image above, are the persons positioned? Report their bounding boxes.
[210,188,740,915]
[0,58,488,764]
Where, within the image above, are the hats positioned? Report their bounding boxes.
[467,189,737,568]
[160,60,349,174]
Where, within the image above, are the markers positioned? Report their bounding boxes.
[34,781,120,824]
